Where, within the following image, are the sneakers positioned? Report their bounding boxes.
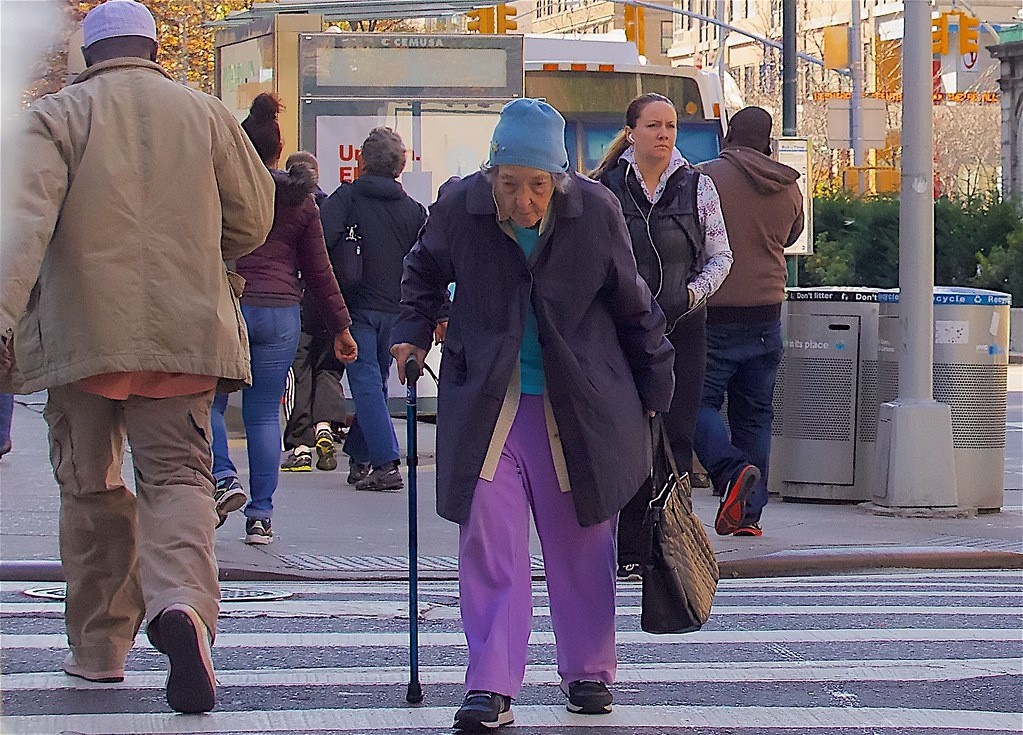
[158,602,216,714]
[714,462,761,534]
[214,478,247,529]
[566,679,612,715]
[347,455,370,484]
[314,429,338,470]
[453,681,515,731]
[63,651,124,683]
[280,450,312,472]
[735,520,760,538]
[244,515,273,544]
[355,462,403,492]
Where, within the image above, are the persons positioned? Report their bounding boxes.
[691,106,804,536]
[318,127,452,491]
[600,93,734,581]
[0,0,276,714]
[210,92,358,544]
[281,151,339,472]
[389,97,675,728]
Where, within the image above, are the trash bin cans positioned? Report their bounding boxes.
[709,287,799,498]
[879,287,1012,515]
[780,285,884,503]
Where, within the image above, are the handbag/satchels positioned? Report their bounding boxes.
[638,411,719,635]
[330,188,363,288]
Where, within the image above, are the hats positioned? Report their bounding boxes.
[487,99,570,174]
[83,1,156,43]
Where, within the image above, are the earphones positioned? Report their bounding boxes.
[628,134,635,144]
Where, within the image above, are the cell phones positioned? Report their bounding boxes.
[763,146,773,156]
